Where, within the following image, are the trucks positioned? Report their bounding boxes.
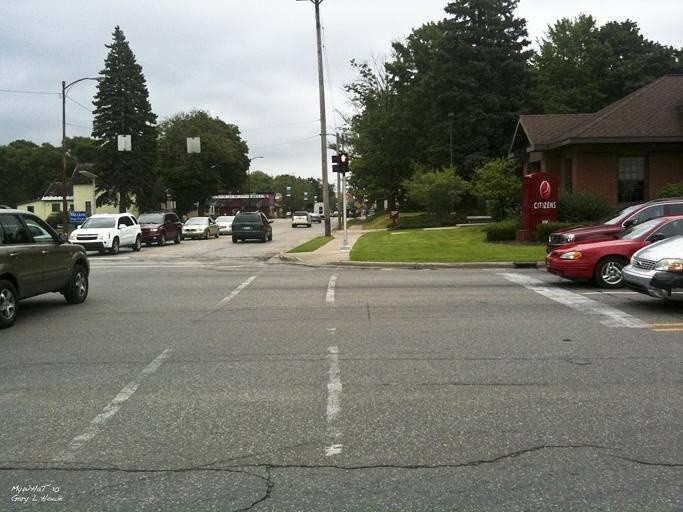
[313,202,330,220]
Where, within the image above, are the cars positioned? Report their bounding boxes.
[309,213,322,223]
[329,208,356,218]
[546,196,682,307]
[2,222,53,241]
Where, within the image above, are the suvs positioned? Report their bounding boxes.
[0,205,93,329]
[291,210,312,227]
[65,209,275,256]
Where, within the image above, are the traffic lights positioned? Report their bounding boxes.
[331,153,341,172]
[338,152,349,174]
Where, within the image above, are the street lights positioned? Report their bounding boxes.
[318,132,347,228]
[248,153,264,211]
[61,77,107,243]
